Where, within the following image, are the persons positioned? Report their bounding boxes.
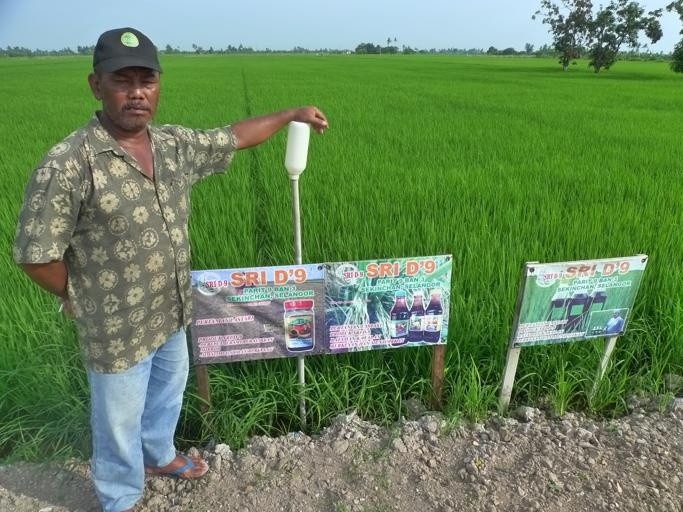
[604,310,625,334]
[11,28,329,512]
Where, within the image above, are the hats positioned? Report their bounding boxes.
[93,27,164,75]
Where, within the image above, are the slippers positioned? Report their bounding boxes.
[146,454,209,478]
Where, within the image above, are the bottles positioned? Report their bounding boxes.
[579,281,607,332]
[408,292,425,342]
[564,288,587,333]
[425,290,443,343]
[549,278,570,331]
[389,291,409,345]
[283,120,311,181]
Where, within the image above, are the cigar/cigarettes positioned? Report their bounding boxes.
[58,304,64,313]
